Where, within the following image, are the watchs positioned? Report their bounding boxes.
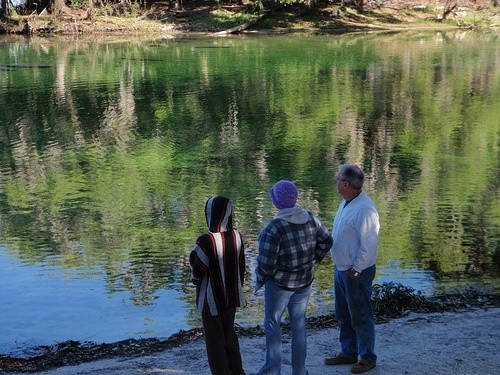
[351,268,360,277]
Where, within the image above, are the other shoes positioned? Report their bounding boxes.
[324,353,359,364]
[351,359,375,373]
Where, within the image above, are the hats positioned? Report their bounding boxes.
[270,180,298,209]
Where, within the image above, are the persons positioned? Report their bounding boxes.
[249,180,334,375]
[325,163,380,373]
[189,195,248,375]
